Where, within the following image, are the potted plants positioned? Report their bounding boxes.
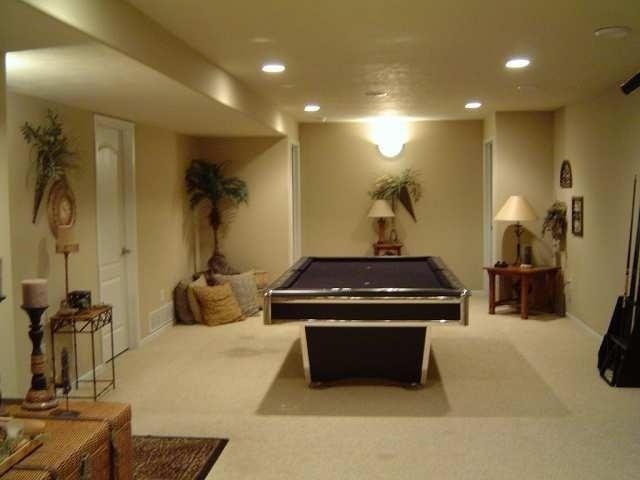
[371,169,422,223]
[185,159,248,285]
[17,106,79,224]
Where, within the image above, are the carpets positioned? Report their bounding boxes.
[132,435,229,480]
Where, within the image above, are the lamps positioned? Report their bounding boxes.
[495,196,538,266]
[368,200,395,244]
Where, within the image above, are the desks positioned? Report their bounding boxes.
[49,306,115,401]
[0,398,131,480]
[488,265,561,319]
[263,256,471,386]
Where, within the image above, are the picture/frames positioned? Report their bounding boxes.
[571,198,583,236]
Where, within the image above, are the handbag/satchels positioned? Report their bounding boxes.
[598,296,639,390]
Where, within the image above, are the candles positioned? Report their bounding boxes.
[21,280,48,307]
[56,225,80,243]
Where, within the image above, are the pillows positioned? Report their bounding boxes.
[175,272,269,327]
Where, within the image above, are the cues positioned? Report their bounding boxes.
[621,175,640,337]
